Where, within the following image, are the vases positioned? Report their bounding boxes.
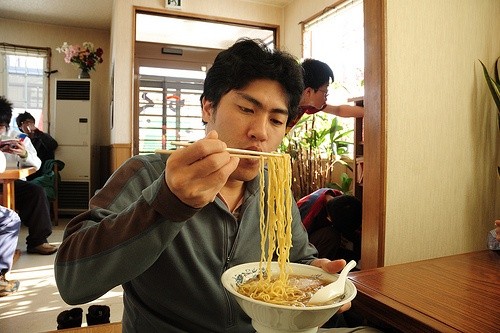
[79,66,90,79]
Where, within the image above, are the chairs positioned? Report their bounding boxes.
[31,160,65,226]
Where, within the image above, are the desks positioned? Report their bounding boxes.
[0,166,36,211]
[348,248,500,333]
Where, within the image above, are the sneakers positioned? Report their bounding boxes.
[0,274,20,296]
[27,242,58,255]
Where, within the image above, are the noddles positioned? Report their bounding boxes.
[237,152,344,307]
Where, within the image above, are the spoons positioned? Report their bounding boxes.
[308,258,357,305]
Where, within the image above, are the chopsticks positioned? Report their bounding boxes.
[154,141,290,160]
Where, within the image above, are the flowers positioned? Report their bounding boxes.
[56,41,103,72]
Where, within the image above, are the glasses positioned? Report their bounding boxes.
[21,120,34,125]
[318,88,329,98]
[0,125,9,129]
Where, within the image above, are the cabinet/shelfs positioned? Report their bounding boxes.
[347,96,364,196]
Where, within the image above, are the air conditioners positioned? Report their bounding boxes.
[53,78,91,180]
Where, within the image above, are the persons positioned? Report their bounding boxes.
[297,187,362,259]
[54,41,351,333]
[0,97,65,297]
[285,58,364,136]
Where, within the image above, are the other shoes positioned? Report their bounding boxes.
[56,307,83,330]
[86,305,111,326]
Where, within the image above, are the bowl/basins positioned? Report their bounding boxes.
[221,261,357,333]
